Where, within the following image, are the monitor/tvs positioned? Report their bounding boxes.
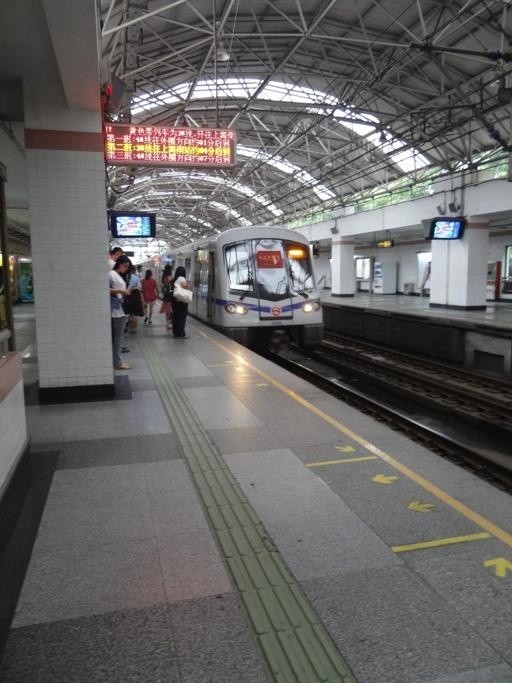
[111,212,156,238]
[430,216,465,240]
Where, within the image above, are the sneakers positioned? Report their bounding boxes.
[121,347,129,353]
[144,317,152,323]
[115,362,129,369]
[174,335,189,339]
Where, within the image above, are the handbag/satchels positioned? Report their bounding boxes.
[173,286,193,303]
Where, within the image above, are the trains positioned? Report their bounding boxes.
[132,226,323,350]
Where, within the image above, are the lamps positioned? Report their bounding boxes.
[379,132,388,142]
[448,203,461,213]
[436,205,446,215]
[213,42,230,61]
[330,227,338,234]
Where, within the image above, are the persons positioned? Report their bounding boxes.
[108,246,190,369]
